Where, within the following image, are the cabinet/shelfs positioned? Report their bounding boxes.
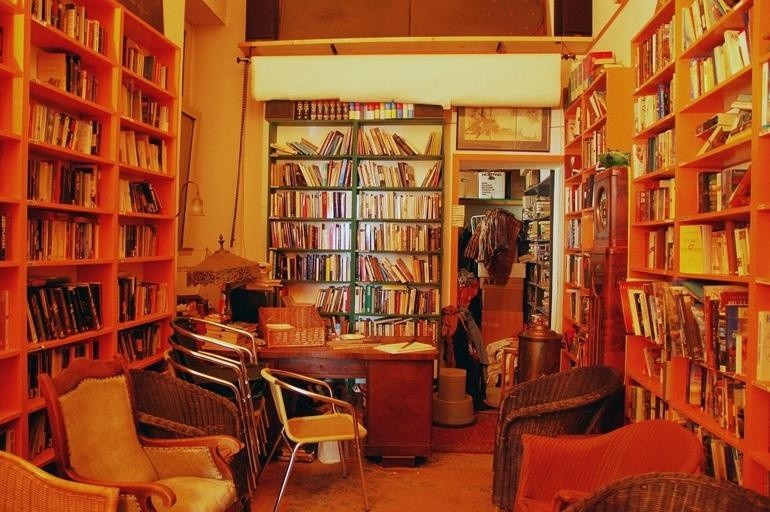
[522,177,552,329]
[117,3,179,371]
[622,1,770,496]
[0,0,27,452]
[558,67,629,373]
[270,121,444,353]
[585,162,629,373]
[27,0,118,468]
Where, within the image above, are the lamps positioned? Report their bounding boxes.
[180,182,208,250]
[186,232,261,322]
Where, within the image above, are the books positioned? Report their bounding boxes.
[761,62,770,132]
[617,1,752,486]
[268,100,444,344]
[1,0,169,401]
[202,314,257,352]
[756,311,770,381]
[522,195,553,325]
[564,51,616,360]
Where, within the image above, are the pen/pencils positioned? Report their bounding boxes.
[401,338,416,349]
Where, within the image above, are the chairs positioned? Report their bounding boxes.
[511,419,707,512]
[489,363,624,512]
[560,470,770,512]
[162,315,268,500]
[260,366,372,512]
[3,451,120,511]
[37,358,250,511]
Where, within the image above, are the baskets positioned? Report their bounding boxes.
[257,307,326,349]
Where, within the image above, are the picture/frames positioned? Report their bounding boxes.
[457,106,550,152]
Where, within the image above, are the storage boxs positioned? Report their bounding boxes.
[478,168,506,202]
[456,170,478,197]
[509,169,527,201]
[520,168,551,190]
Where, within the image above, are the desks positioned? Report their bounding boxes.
[200,335,441,467]
